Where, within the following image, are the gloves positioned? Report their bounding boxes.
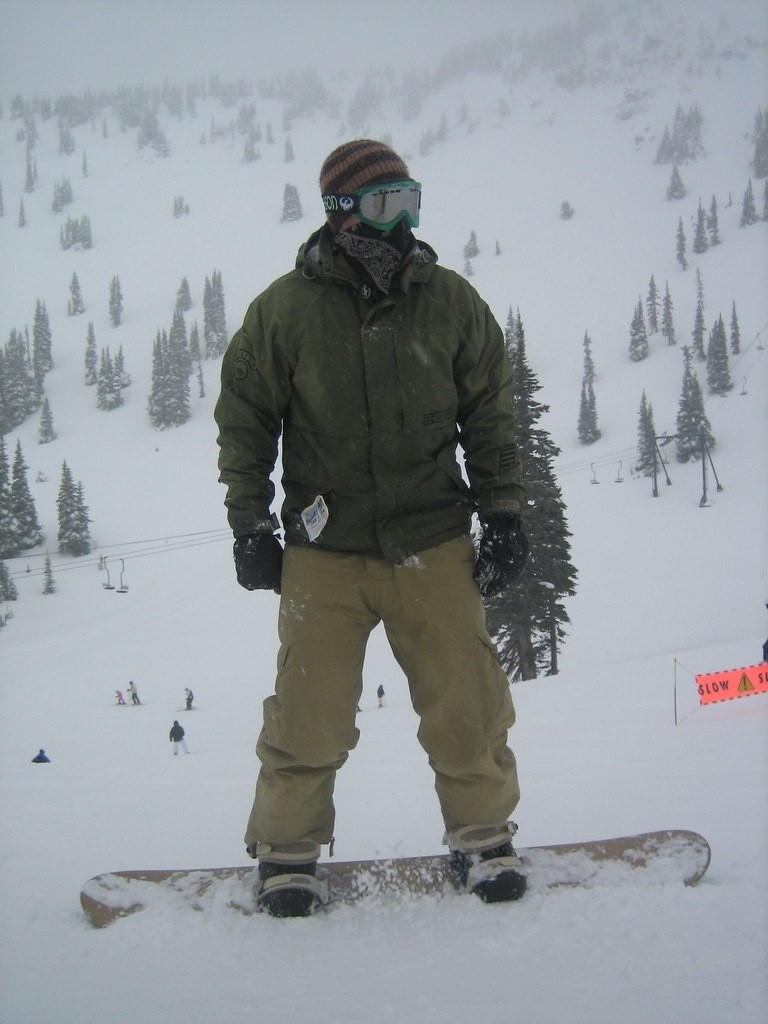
[473,514,530,597]
[233,535,283,597]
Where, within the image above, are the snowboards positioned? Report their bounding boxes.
[75,826,710,930]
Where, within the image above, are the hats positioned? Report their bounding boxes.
[321,139,411,236]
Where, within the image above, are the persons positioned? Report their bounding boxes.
[212,139,534,919]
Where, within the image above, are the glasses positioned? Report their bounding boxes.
[351,179,422,231]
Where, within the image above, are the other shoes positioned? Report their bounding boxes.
[450,851,525,905]
[260,858,318,917]
